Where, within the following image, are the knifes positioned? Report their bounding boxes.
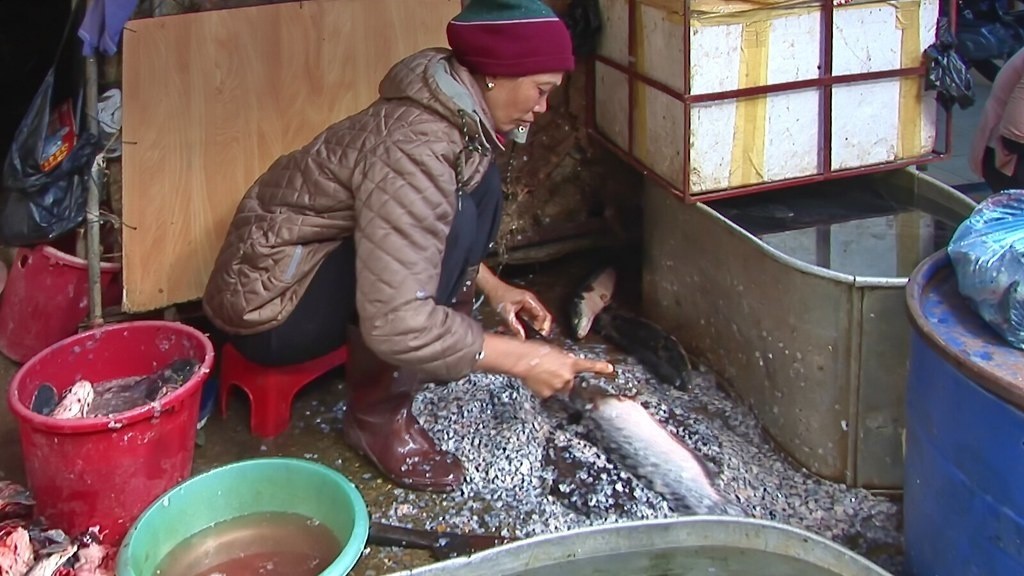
[369,521,521,561]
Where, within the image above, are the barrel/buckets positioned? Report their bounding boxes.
[904,244,1024,576]
[7,320,214,551]
[0,242,121,364]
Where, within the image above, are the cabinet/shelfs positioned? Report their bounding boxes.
[596,0,941,194]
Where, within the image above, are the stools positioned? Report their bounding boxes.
[217,340,346,439]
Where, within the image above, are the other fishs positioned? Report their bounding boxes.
[568,261,616,340]
[566,377,750,518]
[30,358,202,418]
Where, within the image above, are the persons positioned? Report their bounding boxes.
[203,0,615,489]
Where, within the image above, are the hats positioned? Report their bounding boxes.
[446,0,576,77]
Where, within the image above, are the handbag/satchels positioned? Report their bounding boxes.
[0,0,98,246]
[946,186,1024,353]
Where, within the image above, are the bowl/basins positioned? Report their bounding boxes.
[114,457,369,576]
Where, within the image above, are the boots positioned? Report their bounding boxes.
[448,283,476,316]
[343,324,466,491]
